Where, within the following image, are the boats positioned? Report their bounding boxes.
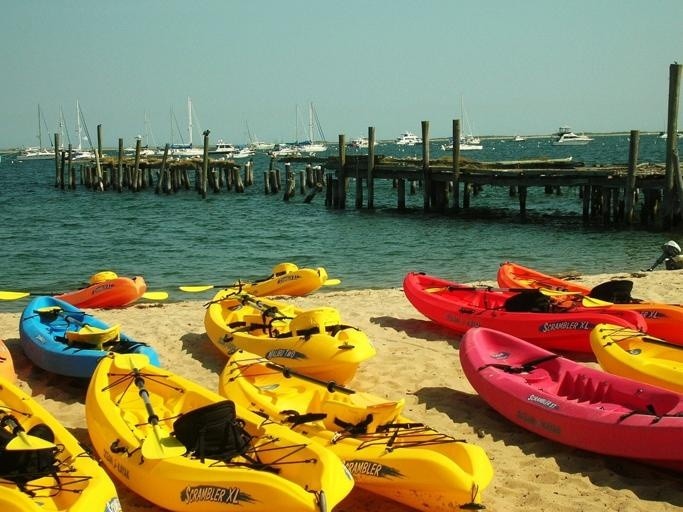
[394,130,422,145]
[438,94,484,152]
[0,356,122,512]
[351,135,379,149]
[52,275,146,308]
[207,100,329,160]
[551,125,594,146]
[587,320,682,394]
[454,327,682,470]
[86,353,355,512]
[216,350,494,511]
[511,135,525,142]
[233,263,326,298]
[402,269,645,348]
[202,287,375,389]
[496,263,682,346]
[17,294,159,377]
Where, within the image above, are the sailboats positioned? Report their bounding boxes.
[121,95,205,157]
[15,94,108,161]
[656,119,683,139]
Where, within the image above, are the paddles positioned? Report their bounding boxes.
[519,279,614,308]
[1,406,57,452]
[179,278,341,293]
[114,353,193,459]
[0,290,168,300]
[424,286,583,296]
[236,354,398,406]
[37,306,119,343]
